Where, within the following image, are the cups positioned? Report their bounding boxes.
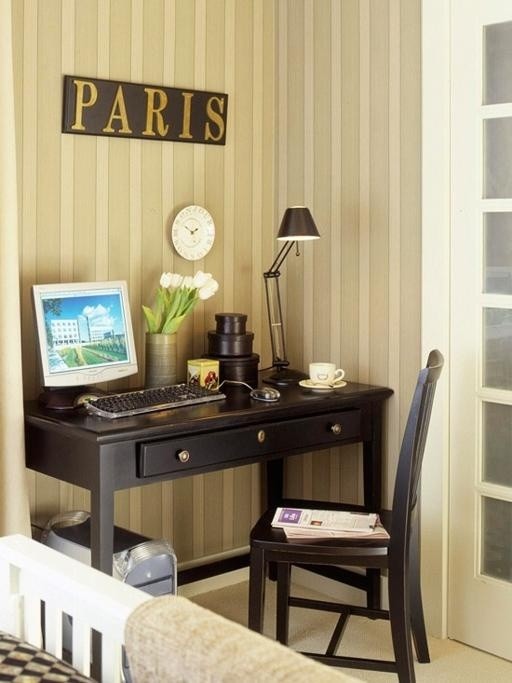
[309,361,345,384]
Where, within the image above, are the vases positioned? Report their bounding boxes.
[144,331,178,388]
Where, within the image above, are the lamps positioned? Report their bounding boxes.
[261,206,321,389]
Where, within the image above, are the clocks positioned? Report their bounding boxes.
[171,205,216,262]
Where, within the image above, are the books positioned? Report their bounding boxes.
[271,505,391,541]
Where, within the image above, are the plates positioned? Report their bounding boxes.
[297,376,349,390]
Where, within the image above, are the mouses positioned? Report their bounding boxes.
[250,387,281,402]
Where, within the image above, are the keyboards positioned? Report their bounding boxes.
[84,383,227,419]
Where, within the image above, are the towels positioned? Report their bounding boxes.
[123,591,358,683]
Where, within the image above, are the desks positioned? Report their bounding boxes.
[24,370,395,621]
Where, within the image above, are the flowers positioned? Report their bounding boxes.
[139,270,219,334]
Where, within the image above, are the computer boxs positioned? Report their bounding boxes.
[39,509,177,598]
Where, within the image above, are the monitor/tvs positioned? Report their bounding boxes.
[33,280,139,409]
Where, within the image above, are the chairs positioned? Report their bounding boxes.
[248,349,445,683]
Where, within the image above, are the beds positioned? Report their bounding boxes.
[1,532,363,683]
